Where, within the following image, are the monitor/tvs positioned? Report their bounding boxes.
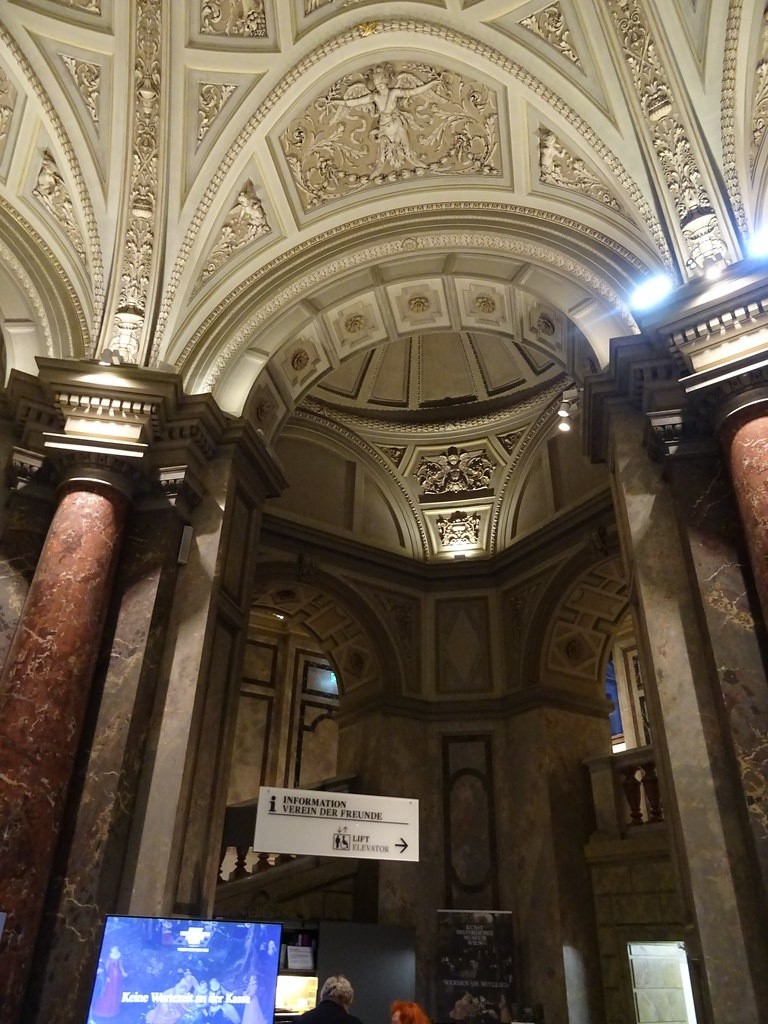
[85,914,284,1024]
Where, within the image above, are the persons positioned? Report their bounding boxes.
[326,68,442,145]
[390,1001,430,1024]
[238,193,266,228]
[293,976,364,1024]
[540,135,566,167]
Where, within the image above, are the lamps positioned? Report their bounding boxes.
[558,415,573,432]
[558,398,574,417]
